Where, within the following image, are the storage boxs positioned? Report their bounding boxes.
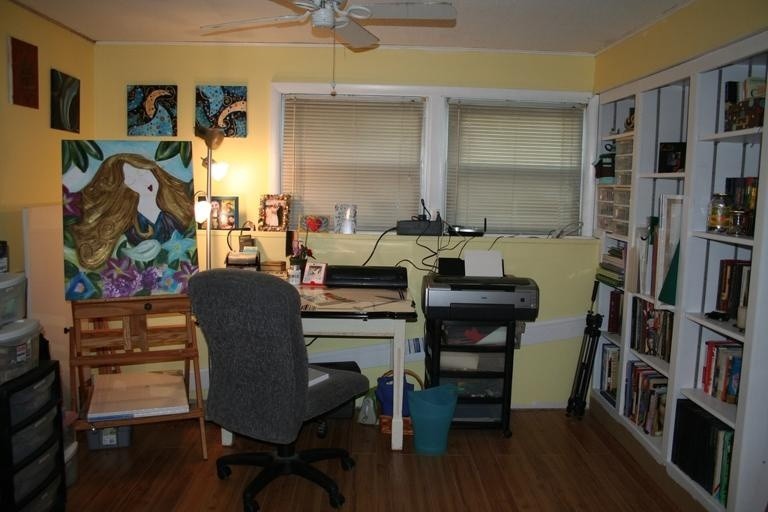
[597,136,633,236]
[87,423,132,451]
[64,411,80,486]
[1,314,41,385]
[0,272,27,327]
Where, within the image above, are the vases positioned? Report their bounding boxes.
[290,256,307,274]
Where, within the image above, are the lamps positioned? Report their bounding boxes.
[309,8,336,30]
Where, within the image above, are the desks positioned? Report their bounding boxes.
[219,286,418,451]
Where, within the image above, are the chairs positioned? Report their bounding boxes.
[189,269,370,511]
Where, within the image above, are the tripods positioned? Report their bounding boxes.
[564,281,604,419]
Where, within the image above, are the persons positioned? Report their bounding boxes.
[266,202,279,226]
[226,215,235,228]
[201,200,219,229]
[276,202,283,226]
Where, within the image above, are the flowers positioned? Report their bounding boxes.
[290,242,314,260]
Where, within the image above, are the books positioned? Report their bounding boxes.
[713,430,735,502]
[608,291,624,335]
[600,343,619,406]
[595,246,625,285]
[702,340,744,403]
[716,259,752,332]
[307,367,329,388]
[631,297,674,363]
[623,360,669,436]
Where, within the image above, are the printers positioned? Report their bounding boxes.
[422,250,539,321]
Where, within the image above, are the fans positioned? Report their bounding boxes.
[200,0,457,50]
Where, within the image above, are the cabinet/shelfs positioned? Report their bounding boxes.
[589,34,768,511]
[424,316,513,438]
[0,360,69,512]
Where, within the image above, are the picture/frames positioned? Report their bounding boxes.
[198,195,239,230]
[659,142,686,172]
[654,191,683,301]
[302,262,328,285]
[257,193,292,232]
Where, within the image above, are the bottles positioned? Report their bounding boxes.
[706,193,731,234]
[287,265,301,286]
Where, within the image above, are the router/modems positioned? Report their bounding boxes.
[449,217,487,238]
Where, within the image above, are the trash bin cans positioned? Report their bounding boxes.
[407,385,459,453]
